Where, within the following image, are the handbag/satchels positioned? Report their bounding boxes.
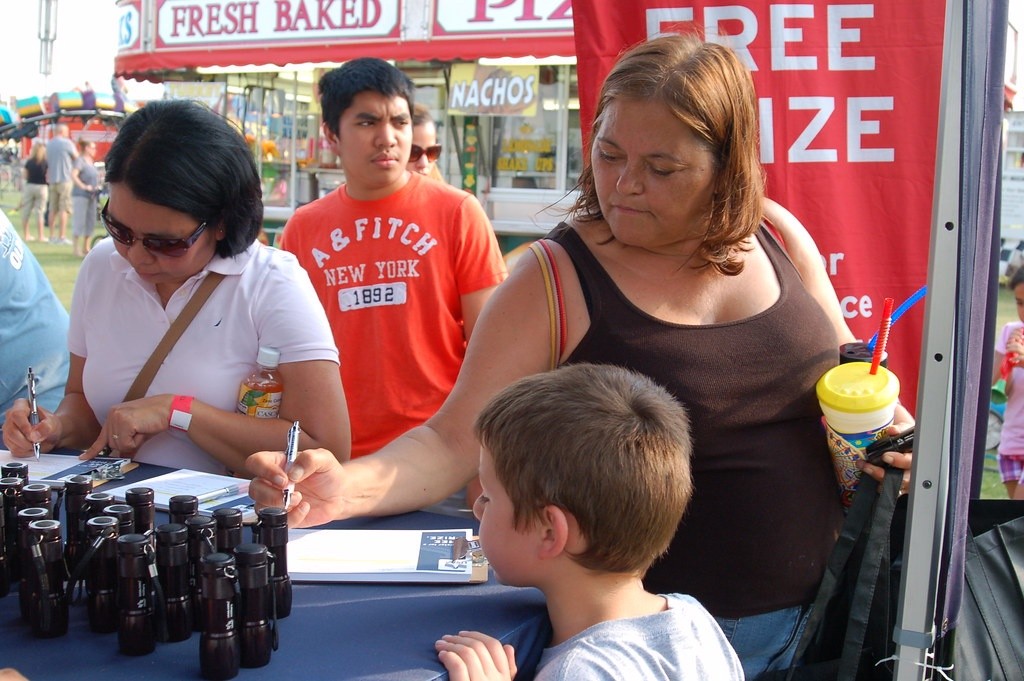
[759,461,1024,681]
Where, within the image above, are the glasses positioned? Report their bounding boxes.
[409,144,442,163]
[99,198,208,258]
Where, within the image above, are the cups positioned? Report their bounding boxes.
[839,344,889,369]
[816,361,899,515]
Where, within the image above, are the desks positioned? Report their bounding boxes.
[0,446,552,681]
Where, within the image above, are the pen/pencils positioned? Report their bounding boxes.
[283,420,301,507]
[27,366,42,461]
[197,482,250,503]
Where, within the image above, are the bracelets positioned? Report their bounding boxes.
[169,395,193,434]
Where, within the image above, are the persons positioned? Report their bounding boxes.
[434,363,747,680]
[991,264,1024,499]
[247,34,915,681]
[279,56,509,459]
[0,204,83,456]
[2,100,352,463]
[0,125,104,256]
[406,103,446,183]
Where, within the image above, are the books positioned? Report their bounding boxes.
[101,468,256,517]
[0,449,131,486]
[287,528,473,582]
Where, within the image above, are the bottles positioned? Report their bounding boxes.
[225,347,282,480]
